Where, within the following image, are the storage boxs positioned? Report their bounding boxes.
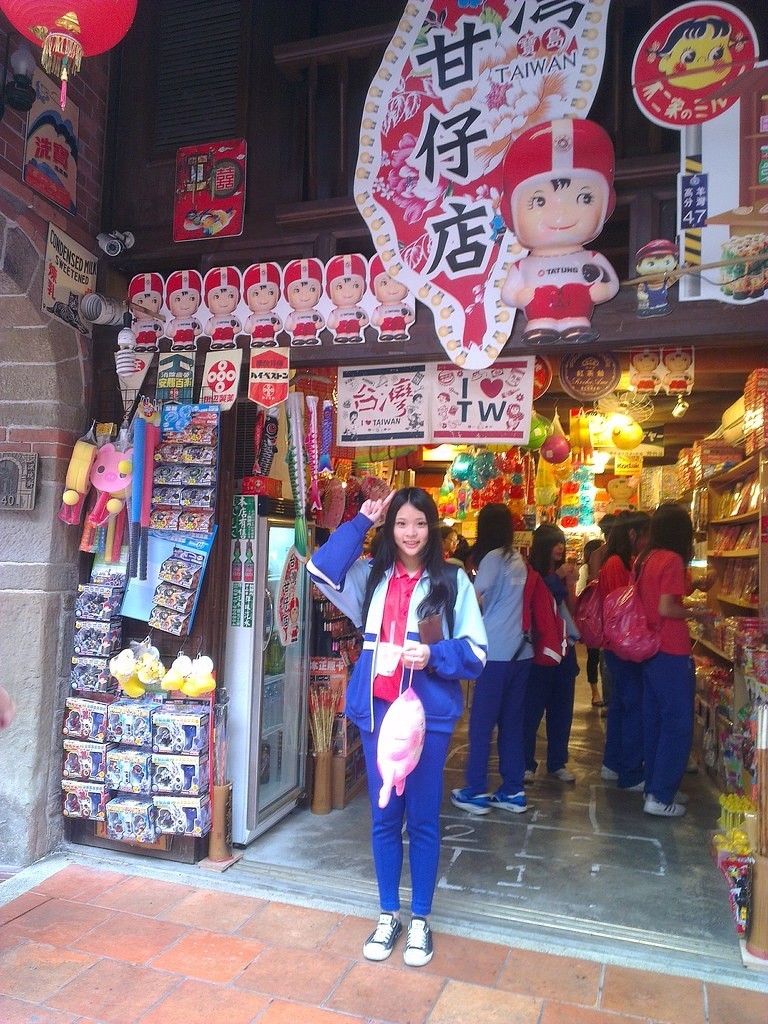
[63,548,211,844]
[307,658,368,810]
[677,369,768,491]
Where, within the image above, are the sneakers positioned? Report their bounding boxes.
[451,788,491,815]
[643,791,689,818]
[363,913,403,961]
[403,916,434,967]
[491,791,528,814]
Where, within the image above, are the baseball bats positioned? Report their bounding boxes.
[140,423,156,580]
[128,417,146,578]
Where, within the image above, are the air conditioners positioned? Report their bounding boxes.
[721,395,746,447]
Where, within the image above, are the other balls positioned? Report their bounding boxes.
[119,461,132,474]
[106,498,122,514]
[62,490,79,506]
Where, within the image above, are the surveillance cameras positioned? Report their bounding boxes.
[96,232,122,256]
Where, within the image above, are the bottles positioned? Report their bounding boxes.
[232,541,242,581]
[262,585,286,675]
[244,541,254,582]
[260,737,270,783]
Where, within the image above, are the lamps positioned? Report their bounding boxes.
[0,32,37,119]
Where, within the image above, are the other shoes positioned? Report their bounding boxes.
[627,780,645,791]
[553,769,576,782]
[592,698,602,706]
[601,765,619,780]
[524,770,536,784]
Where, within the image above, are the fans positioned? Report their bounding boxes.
[594,387,655,423]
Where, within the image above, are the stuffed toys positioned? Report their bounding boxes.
[376,687,426,808]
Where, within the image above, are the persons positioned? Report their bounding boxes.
[630,344,694,395]
[443,502,711,817]
[305,487,488,966]
[604,473,637,515]
[130,253,417,351]
[501,118,621,341]
[634,241,692,318]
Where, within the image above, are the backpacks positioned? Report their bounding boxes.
[511,563,568,666]
[574,578,603,648]
[601,549,667,662]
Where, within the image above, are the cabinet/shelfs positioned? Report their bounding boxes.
[675,448,768,816]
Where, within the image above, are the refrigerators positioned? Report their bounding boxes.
[225,491,312,850]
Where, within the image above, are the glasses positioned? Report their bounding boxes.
[445,538,459,544]
